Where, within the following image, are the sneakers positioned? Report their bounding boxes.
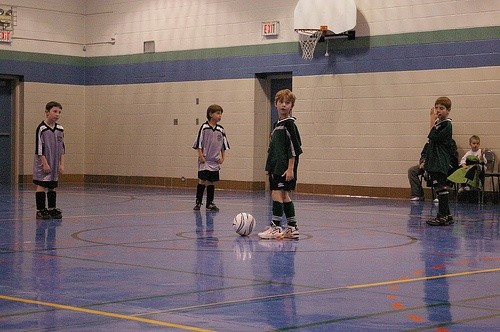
[193,202,203,211]
[47,208,62,219]
[257,221,284,239]
[36,208,52,219]
[282,224,299,238]
[426,213,454,225]
[205,202,219,212]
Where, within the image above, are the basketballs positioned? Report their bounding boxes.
[233,213,257,236]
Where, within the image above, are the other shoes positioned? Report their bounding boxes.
[410,196,425,202]
[458,186,471,193]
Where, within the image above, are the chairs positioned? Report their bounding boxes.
[421,149,500,204]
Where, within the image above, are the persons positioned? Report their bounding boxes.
[408,139,459,203]
[447,135,488,193]
[258,89,303,240]
[419,97,454,225]
[32,101,65,220]
[192,104,232,210]
